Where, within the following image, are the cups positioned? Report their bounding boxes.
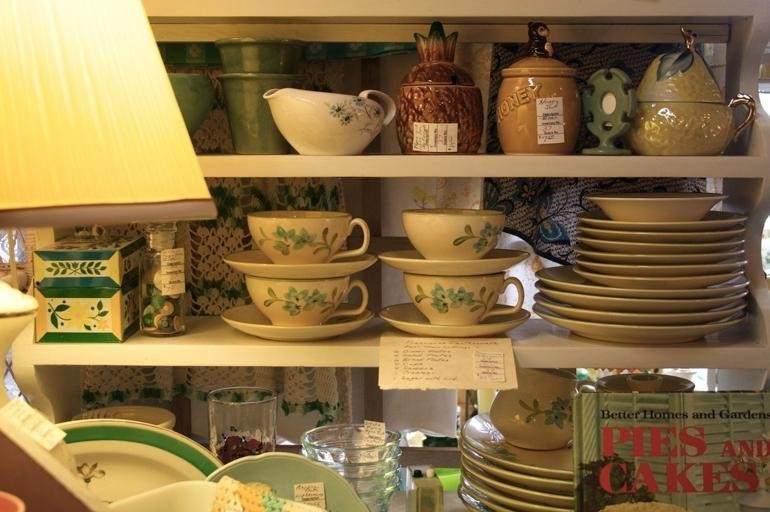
[242,274,369,325]
[262,87,396,155]
[210,386,278,460]
[401,271,524,324]
[402,208,505,259]
[248,207,368,264]
[490,366,598,452]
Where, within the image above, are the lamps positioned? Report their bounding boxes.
[0,0,218,288]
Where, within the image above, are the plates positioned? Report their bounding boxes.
[222,303,375,340]
[24,420,225,496]
[221,249,379,278]
[204,451,371,512]
[534,211,752,343]
[583,193,730,223]
[458,412,574,511]
[379,302,532,335]
[383,249,531,275]
[105,479,218,511]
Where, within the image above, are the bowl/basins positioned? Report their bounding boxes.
[217,72,301,154]
[301,424,403,512]
[167,70,218,139]
[212,35,292,72]
